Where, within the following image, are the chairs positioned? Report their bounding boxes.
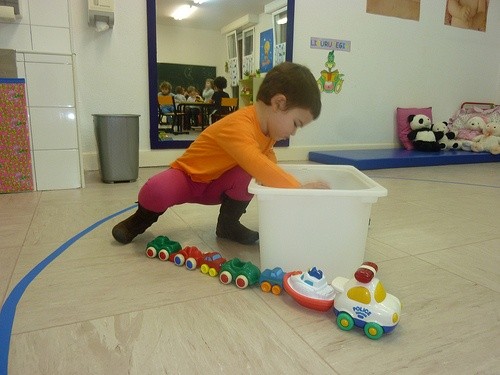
[157,96,237,135]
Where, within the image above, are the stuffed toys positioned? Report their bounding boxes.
[453,115,500,155]
[406,113,444,150]
[433,121,459,149]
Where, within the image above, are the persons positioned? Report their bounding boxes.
[447,0,486,30]
[208,77,230,125]
[202,78,215,101]
[111,63,331,245]
[157,81,176,123]
[174,85,203,126]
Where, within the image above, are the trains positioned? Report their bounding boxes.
[144,234,402,341]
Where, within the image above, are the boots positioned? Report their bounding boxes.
[112,201,165,244]
[216,191,259,244]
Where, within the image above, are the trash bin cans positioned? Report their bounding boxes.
[89,112,142,184]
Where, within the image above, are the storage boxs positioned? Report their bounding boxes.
[247,164,388,274]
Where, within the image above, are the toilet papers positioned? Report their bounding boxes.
[87,0,115,33]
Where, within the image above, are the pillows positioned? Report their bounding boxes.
[396,107,434,150]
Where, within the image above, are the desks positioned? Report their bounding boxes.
[177,100,212,131]
